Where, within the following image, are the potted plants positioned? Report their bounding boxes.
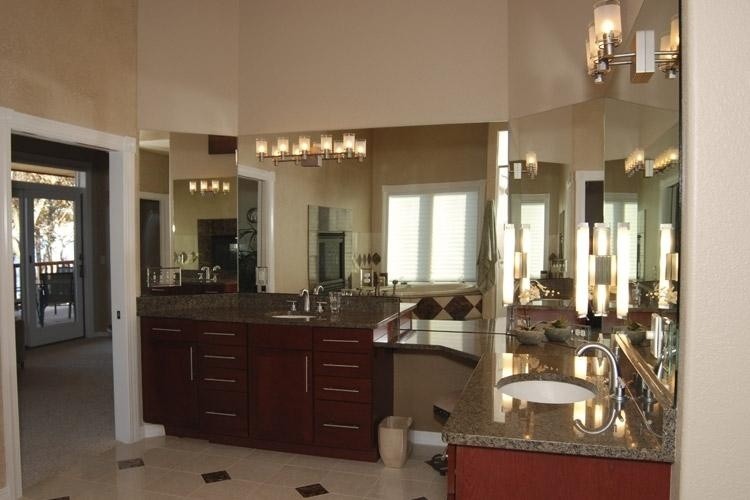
[514,327,546,345]
[543,319,571,343]
[621,321,647,345]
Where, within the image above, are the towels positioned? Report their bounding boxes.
[477,199,503,295]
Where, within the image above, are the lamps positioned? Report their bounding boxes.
[623,148,680,178]
[650,148,679,177]
[585,0,645,85]
[256,133,369,166]
[189,180,230,201]
[507,149,541,180]
[624,149,649,177]
[645,14,680,79]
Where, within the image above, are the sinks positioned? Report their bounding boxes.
[265,311,321,318]
[497,373,598,404]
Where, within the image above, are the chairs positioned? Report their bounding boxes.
[36,267,76,328]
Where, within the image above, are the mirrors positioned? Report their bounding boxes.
[136,94,681,407]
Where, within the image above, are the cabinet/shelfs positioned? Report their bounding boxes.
[141,316,399,463]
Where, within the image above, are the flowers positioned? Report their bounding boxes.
[514,280,560,330]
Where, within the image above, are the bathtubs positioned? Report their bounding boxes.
[381,282,476,296]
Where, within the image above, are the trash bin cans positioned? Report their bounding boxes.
[378,415,415,468]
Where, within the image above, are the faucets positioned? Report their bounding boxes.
[201,266,210,280]
[573,407,617,436]
[375,282,384,296]
[299,288,310,312]
[313,286,324,295]
[655,347,678,381]
[213,266,221,273]
[576,343,618,393]
